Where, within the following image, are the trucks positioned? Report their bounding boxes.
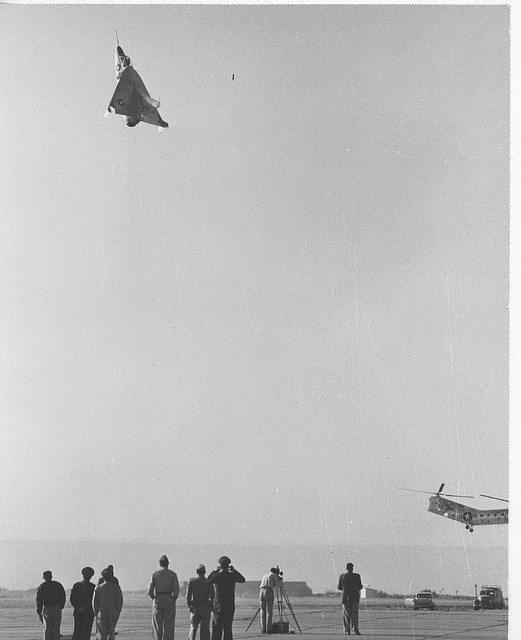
[473,585,503,611]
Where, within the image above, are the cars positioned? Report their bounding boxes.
[411,592,435,611]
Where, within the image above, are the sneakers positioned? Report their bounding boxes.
[354,631,361,635]
[345,632,349,634]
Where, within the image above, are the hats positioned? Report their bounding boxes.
[160,555,168,562]
[347,563,354,569]
[82,567,94,576]
[218,557,231,564]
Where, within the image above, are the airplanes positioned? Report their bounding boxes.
[105,29,169,131]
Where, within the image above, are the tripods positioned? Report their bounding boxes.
[244,583,303,636]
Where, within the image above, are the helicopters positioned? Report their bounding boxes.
[396,483,508,532]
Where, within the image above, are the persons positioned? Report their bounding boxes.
[205,556,245,640]
[258,568,283,634]
[92,568,124,640]
[69,566,97,640]
[96,564,120,635]
[185,564,215,640]
[36,570,66,640]
[337,562,362,636]
[147,555,179,639]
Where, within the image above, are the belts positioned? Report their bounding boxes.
[261,587,269,588]
[156,592,172,595]
